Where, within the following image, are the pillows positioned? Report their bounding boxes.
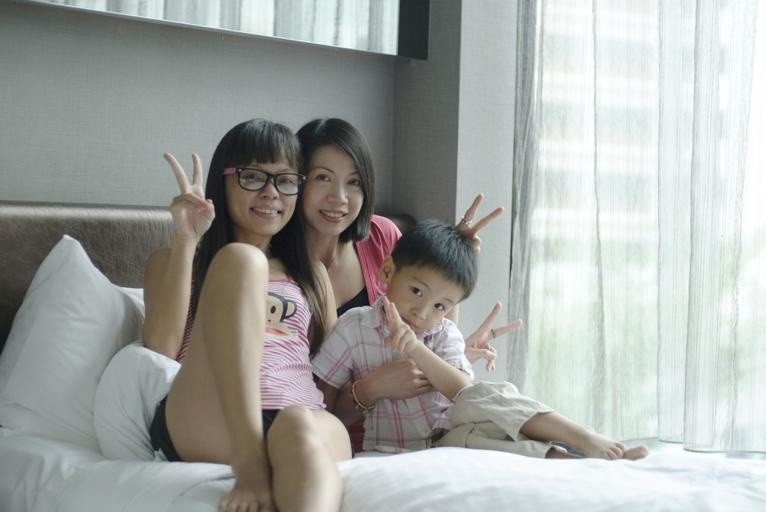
[92,337,183,462]
[0,233,146,456]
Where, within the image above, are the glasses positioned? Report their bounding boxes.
[223,167,306,195]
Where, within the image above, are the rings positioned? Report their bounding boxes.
[460,216,473,225]
[490,328,498,340]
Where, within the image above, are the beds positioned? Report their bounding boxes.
[1,198,766,512]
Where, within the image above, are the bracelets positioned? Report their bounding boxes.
[351,378,376,410]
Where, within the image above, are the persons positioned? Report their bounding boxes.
[295,119,506,452]
[142,118,523,511]
[310,220,648,461]
